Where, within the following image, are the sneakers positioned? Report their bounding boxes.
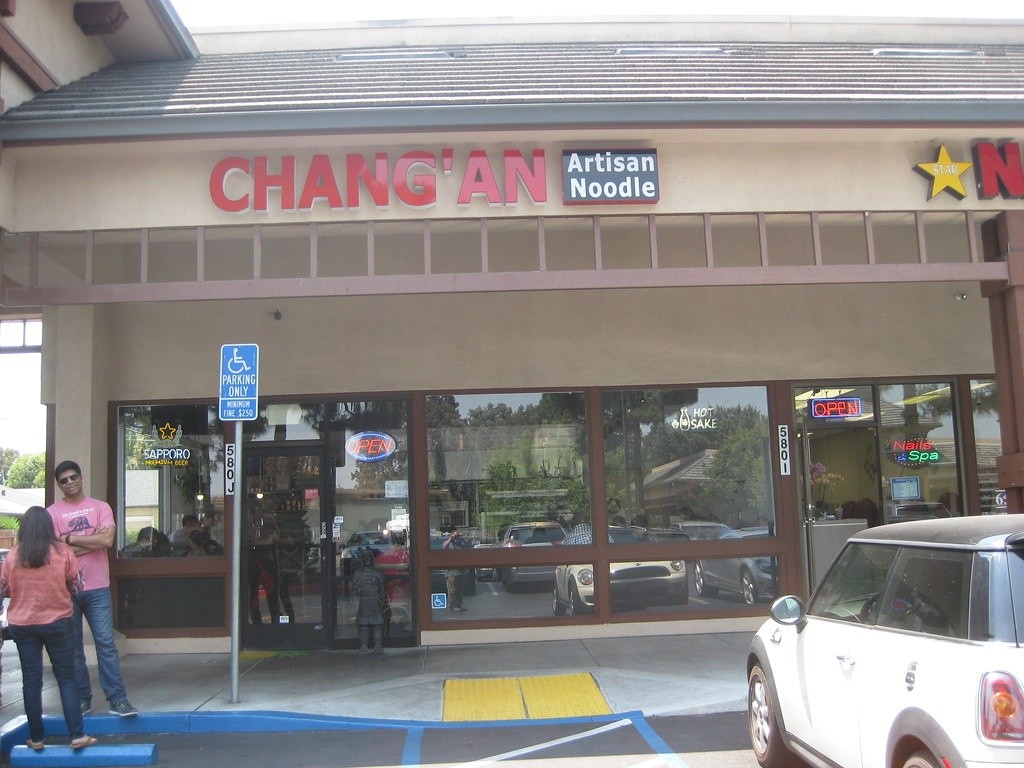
[80,703,92,718]
[109,701,138,716]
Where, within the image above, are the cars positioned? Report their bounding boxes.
[553,526,689,616]
[426,535,475,596]
[670,521,732,543]
[472,543,499,582]
[339,530,411,602]
[747,511,1024,768]
[492,521,572,590]
[694,526,779,607]
[887,502,953,524]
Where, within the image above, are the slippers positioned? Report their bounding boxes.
[27,739,44,749]
[69,736,98,748]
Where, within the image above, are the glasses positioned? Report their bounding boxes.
[59,473,79,485]
[454,535,459,538]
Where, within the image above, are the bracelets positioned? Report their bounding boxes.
[66,534,71,545]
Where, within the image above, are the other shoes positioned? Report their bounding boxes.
[459,606,466,611]
[449,607,461,611]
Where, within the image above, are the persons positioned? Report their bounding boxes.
[118,510,221,626]
[0,506,98,748]
[45,460,137,716]
[444,530,467,613]
[242,499,295,623]
[348,546,389,654]
